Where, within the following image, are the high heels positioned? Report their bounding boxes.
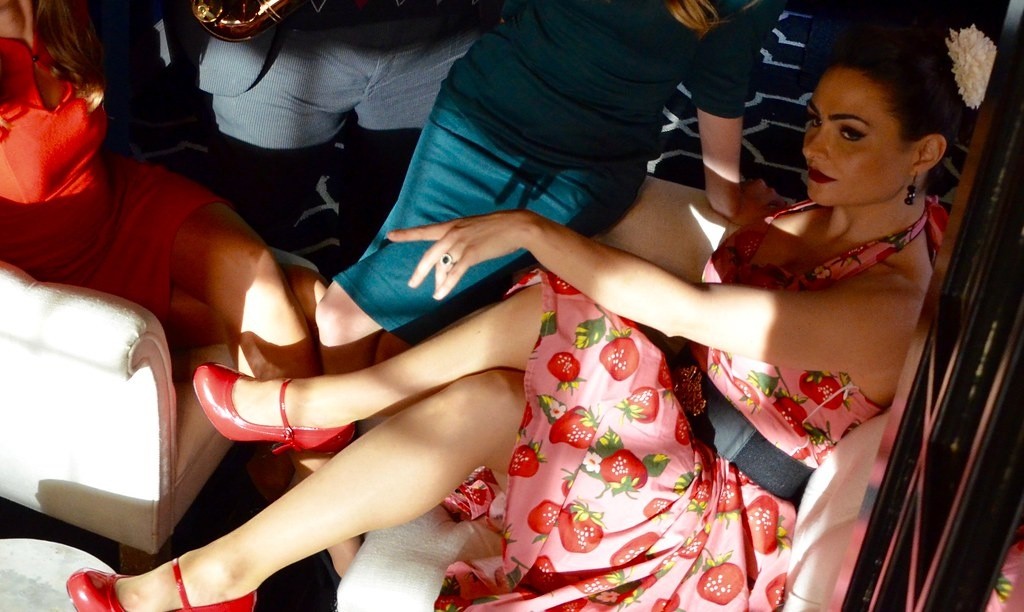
[67,557,257,612]
[193,363,355,456]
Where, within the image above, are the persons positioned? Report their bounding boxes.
[247,0,783,506]
[198,0,505,265]
[0,0,363,578]
[66,25,998,612]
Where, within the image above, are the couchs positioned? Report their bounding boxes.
[335,174,891,612]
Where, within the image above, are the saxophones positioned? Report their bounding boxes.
[186,0,295,43]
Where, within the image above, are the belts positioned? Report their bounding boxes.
[671,347,816,503]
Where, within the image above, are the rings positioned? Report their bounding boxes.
[441,253,455,265]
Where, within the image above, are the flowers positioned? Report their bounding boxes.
[944,23,998,109]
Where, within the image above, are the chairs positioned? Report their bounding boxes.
[0,245,320,576]
